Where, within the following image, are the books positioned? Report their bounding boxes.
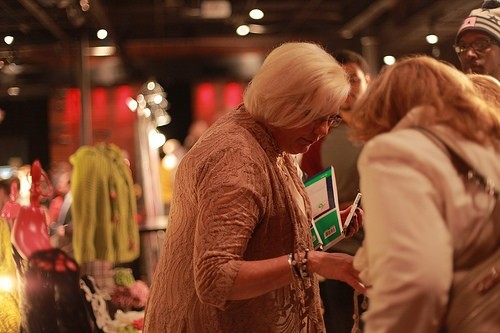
[304,163,345,252]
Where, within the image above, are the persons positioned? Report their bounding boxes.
[141,41,365,333]
[294,49,371,333]
[347,0,500,333]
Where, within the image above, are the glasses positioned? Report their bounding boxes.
[453,38,500,53]
[306,107,342,128]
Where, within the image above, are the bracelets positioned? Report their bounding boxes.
[288,248,309,281]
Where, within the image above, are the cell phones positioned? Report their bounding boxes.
[343,193,362,235]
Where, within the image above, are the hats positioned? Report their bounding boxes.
[455,0,500,43]
